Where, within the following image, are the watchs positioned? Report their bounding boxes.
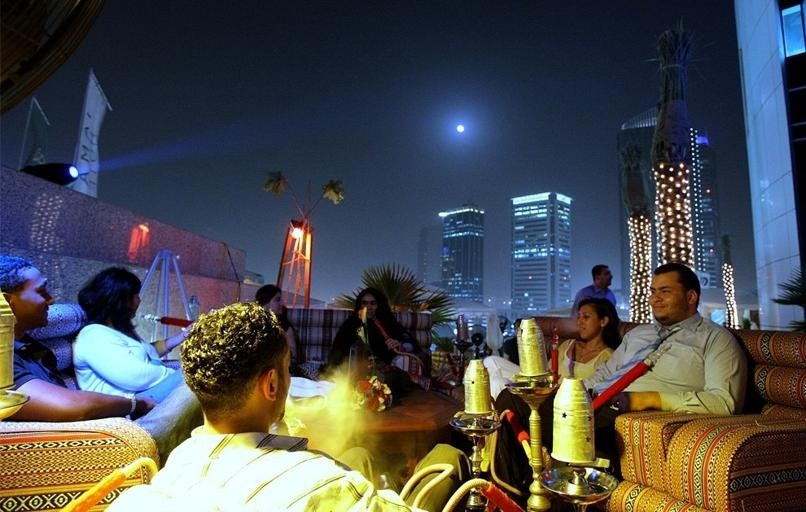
[124,393,137,414]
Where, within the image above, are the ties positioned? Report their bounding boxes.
[592,329,670,396]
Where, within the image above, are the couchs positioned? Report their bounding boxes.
[0,303,159,512]
[530,316,805,512]
[285,308,432,389]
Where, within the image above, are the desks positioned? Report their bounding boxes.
[360,387,457,488]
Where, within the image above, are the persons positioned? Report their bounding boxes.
[329,287,422,384]
[494,262,750,512]
[0,255,204,471]
[71,264,198,405]
[570,264,617,316]
[482,294,622,402]
[147,298,412,512]
[254,282,298,362]
